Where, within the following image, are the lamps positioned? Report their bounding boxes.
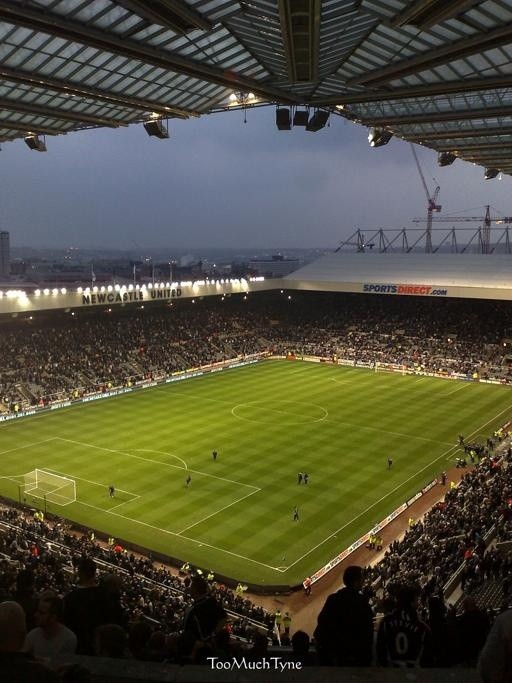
[275,104,330,132]
[436,151,457,168]
[483,166,499,180]
[368,126,395,147]
[142,119,170,140]
[22,135,47,153]
